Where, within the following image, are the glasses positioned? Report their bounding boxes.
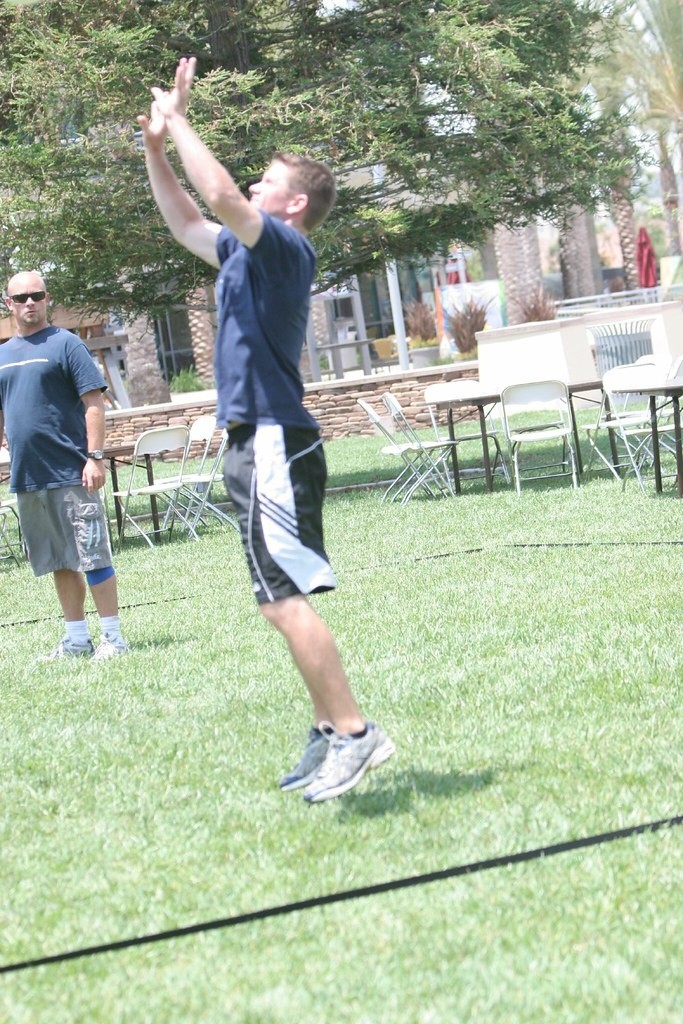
[8,291,48,304]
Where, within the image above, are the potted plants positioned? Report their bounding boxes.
[0,498,26,569]
[399,297,440,369]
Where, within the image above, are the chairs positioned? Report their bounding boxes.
[583,352,683,499]
[112,415,243,558]
[501,379,582,496]
[356,391,460,507]
[424,382,511,498]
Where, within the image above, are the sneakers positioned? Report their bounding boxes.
[36,635,95,667]
[280,724,335,792]
[304,722,395,804]
[89,635,127,666]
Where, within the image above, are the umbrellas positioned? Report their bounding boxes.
[448,253,470,284]
[636,227,656,302]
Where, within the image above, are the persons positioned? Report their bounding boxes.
[0,270,129,666]
[611,274,632,307]
[136,56,397,803]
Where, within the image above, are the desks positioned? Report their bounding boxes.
[100,438,162,545]
[422,371,624,496]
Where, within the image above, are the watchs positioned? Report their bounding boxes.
[87,450,106,460]
